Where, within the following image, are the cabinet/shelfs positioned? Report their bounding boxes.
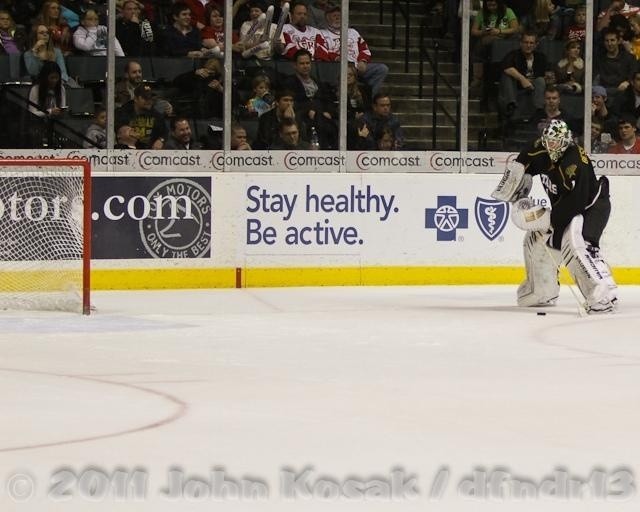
[477,128,488,151]
[49,97,58,114]
[0,0,568,151]
[310,126,320,150]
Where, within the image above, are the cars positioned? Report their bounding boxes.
[60,106,72,115]
[263,91,275,107]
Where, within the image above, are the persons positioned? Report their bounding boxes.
[491,120,617,314]
[0,0,640,154]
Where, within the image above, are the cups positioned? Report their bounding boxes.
[38,31,50,35]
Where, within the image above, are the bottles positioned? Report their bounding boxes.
[134,84,157,99]
[324,4,341,15]
[592,85,608,98]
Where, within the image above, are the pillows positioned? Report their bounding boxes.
[541,118,573,164]
